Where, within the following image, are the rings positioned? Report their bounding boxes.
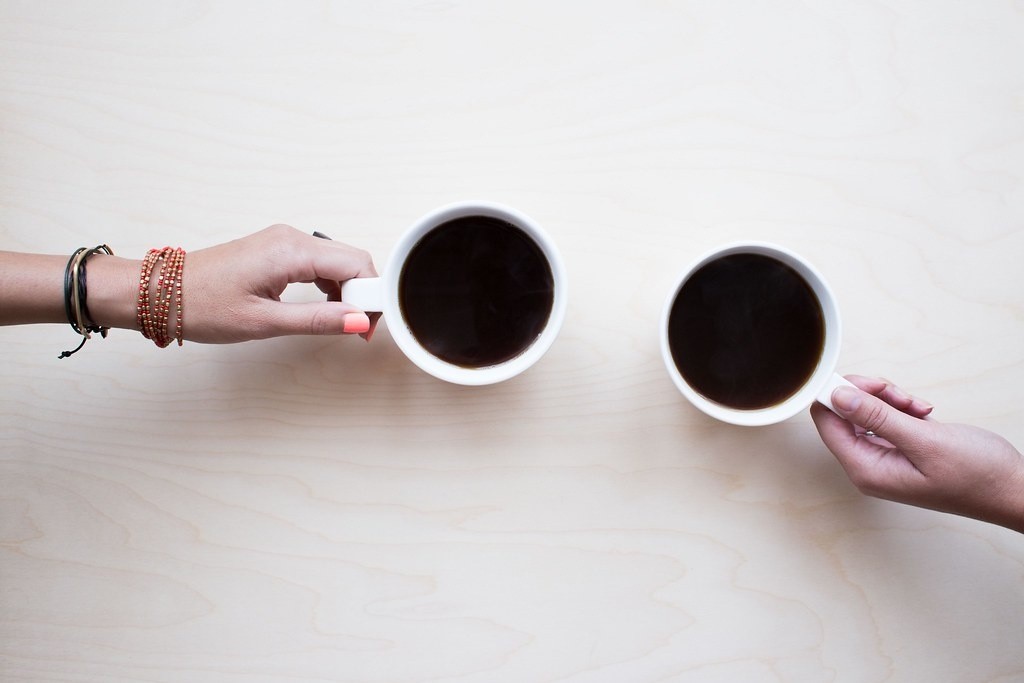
[866,431,875,435]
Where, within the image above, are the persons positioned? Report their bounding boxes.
[0,224,383,345]
[809,375,1024,535]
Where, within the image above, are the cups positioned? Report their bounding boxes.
[659,242,860,426]
[341,201,568,388]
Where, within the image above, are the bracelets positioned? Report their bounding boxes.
[137,245,186,349]
[57,244,114,359]
